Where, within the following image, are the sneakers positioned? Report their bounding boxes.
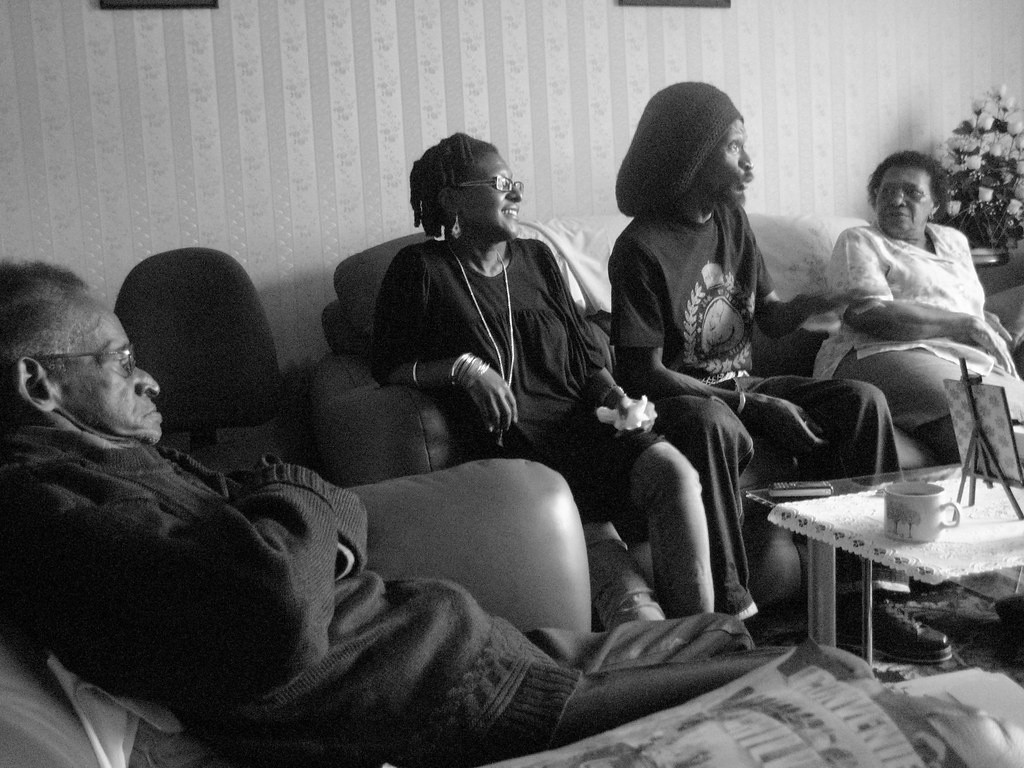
[837,597,953,662]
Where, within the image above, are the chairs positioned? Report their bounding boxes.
[113,248,285,472]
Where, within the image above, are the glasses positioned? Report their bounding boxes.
[458,175,524,194]
[38,344,136,374]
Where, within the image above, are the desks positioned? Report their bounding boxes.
[746,462,1024,682]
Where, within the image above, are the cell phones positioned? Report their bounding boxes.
[769,481,834,497]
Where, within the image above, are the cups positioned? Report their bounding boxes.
[883,480,962,544]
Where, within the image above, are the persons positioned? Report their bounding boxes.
[0,260,876,768]
[370,133,714,631]
[608,83,954,665]
[812,149,1024,465]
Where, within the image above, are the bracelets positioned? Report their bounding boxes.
[411,359,421,390]
[449,351,490,388]
[737,392,745,414]
[601,385,624,405]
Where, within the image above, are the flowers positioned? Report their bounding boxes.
[942,85,1024,248]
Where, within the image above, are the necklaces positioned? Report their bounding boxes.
[447,243,515,448]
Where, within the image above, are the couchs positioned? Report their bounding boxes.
[0,202,1024,768]
